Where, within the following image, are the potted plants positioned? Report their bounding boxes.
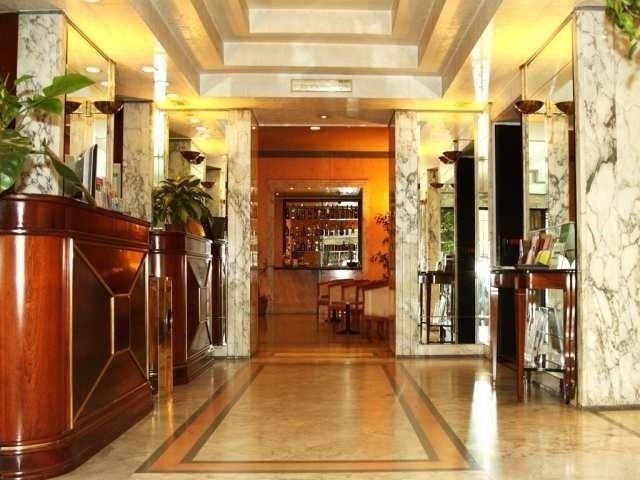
[152,172,215,236]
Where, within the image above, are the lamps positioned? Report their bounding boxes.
[438,156,456,164]
[512,99,548,117]
[549,99,573,118]
[180,150,200,163]
[442,150,463,161]
[89,99,124,117]
[201,181,215,188]
[65,101,88,116]
[195,156,205,164]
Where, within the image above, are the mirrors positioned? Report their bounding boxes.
[418,110,490,346]
[521,13,576,398]
[162,110,230,351]
[61,21,116,206]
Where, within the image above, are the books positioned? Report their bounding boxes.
[548,236,569,270]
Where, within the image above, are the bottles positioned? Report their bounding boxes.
[325,221,355,235]
[326,203,358,219]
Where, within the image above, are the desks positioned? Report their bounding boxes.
[418,272,455,344]
[525,270,576,402]
[489,266,571,405]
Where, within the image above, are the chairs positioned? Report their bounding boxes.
[316,278,391,343]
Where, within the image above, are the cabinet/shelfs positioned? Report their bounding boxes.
[284,218,359,268]
